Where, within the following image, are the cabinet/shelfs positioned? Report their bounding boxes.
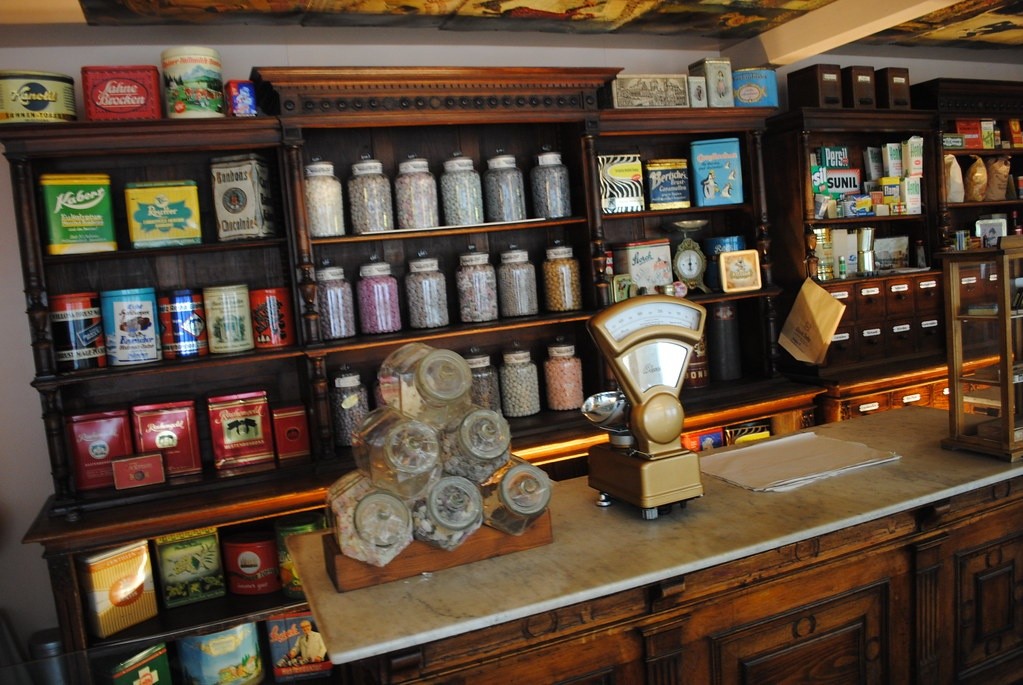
[763,107,1023,425]
[0,67,831,685]
[933,235,1023,464]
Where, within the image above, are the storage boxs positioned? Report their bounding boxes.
[224,80,258,118]
[597,137,744,214]
[100,288,162,366]
[597,57,736,109]
[608,236,672,293]
[810,134,924,219]
[942,119,1023,149]
[267,611,334,683]
[77,527,225,642]
[39,153,277,255]
[68,385,309,490]
[82,66,162,122]
[92,642,171,685]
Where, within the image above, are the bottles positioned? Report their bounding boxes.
[684,301,743,389]
[301,147,583,449]
